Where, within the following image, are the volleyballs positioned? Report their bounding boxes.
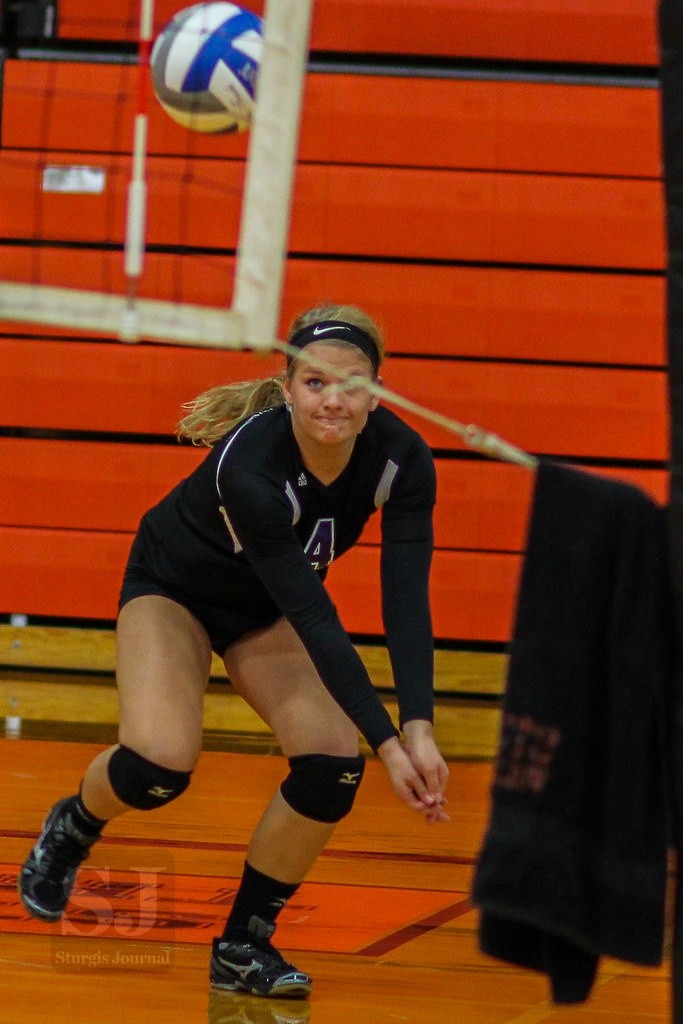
[150,4,264,134]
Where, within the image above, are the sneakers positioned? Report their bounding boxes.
[209,936,313,999]
[17,795,104,923]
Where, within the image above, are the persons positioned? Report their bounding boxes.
[20,305,448,999]
